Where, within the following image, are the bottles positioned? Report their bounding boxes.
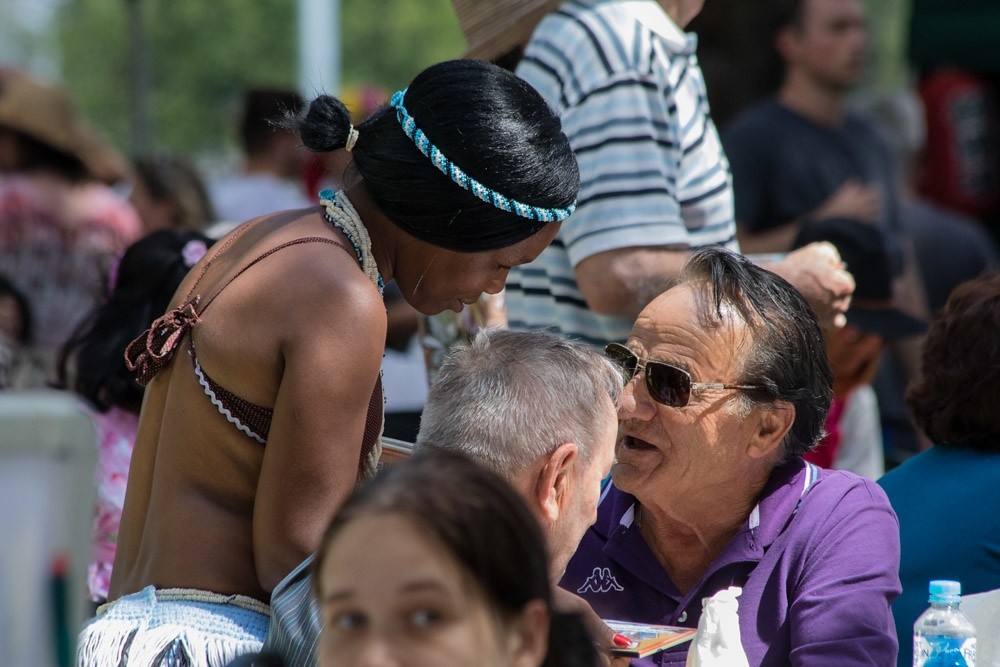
[912,579,980,667]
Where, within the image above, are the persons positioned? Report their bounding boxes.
[0,0,999,667]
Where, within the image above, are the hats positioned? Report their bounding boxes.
[802,221,928,340]
[1,66,125,181]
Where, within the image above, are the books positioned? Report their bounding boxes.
[596,619,698,658]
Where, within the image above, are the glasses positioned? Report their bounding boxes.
[605,341,760,408]
